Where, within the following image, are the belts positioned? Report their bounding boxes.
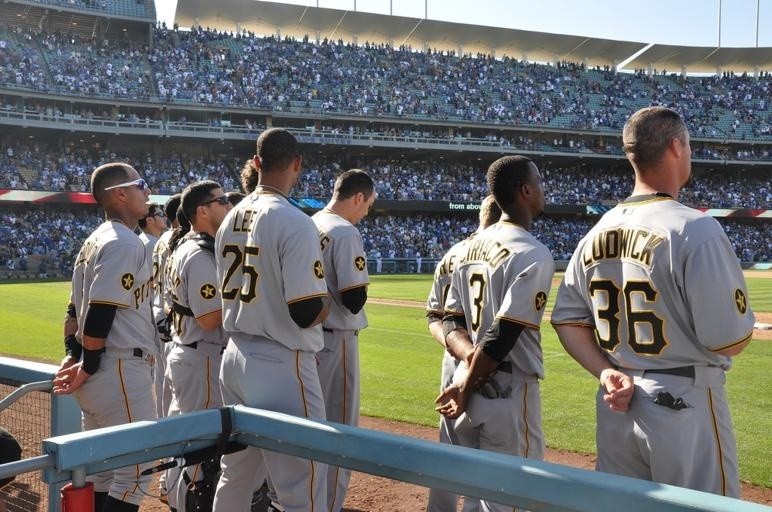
[186,341,226,352]
[320,326,358,335]
[644,365,695,379]
[102,346,144,358]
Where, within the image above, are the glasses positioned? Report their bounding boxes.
[202,195,229,204]
[104,179,146,191]
[155,212,164,217]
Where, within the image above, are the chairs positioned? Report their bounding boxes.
[0,0,771,283]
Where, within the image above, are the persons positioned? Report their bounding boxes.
[484,132,635,259]
[0,105,254,279]
[213,129,331,511]
[308,168,376,511]
[434,155,554,512]
[51,162,161,512]
[290,124,492,274]
[1,7,155,130]
[427,194,501,512]
[552,106,755,501]
[147,18,392,117]
[385,44,604,133]
[137,159,258,511]
[676,143,770,270]
[591,62,771,141]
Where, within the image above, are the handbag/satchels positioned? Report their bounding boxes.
[185,466,272,511]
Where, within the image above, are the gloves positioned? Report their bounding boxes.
[473,374,513,398]
[653,390,689,410]
